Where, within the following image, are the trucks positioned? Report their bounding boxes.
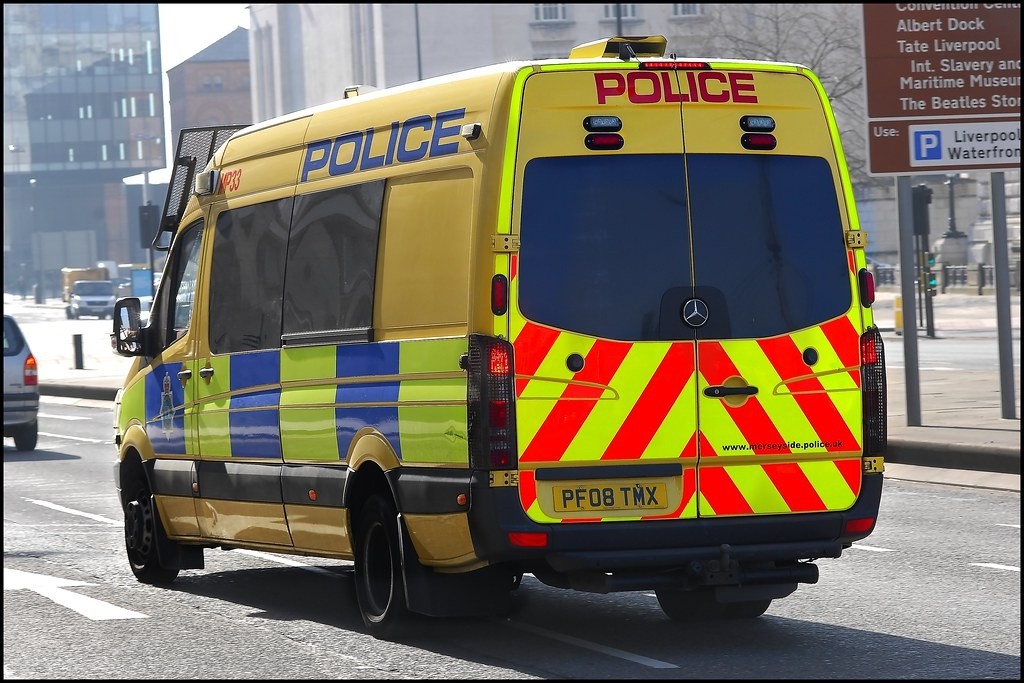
[61,267,116,320]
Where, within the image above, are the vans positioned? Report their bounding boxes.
[109,36,888,641]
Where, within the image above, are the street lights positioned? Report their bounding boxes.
[942,173,968,238]
[131,132,156,298]
[10,148,27,299]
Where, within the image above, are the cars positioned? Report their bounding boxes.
[118,273,162,297]
[4,315,39,451]
